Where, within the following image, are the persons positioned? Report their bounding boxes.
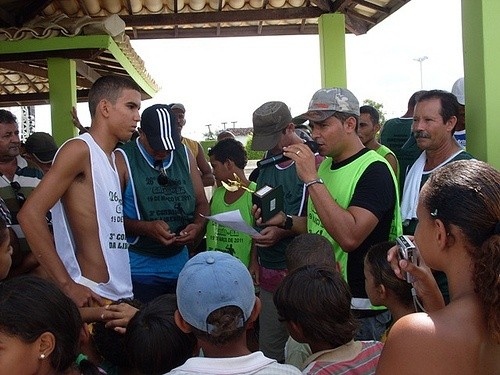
[0,76,500,375]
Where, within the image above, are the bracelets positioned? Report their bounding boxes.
[303,176,326,189]
[278,212,293,232]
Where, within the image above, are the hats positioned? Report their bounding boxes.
[140,104,182,151]
[452,77,465,106]
[169,104,185,113]
[25,132,58,163]
[217,130,235,142]
[292,87,360,124]
[251,102,292,151]
[176,251,256,332]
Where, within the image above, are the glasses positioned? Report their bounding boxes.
[153,158,169,188]
[10,181,26,206]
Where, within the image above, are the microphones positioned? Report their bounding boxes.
[257,141,318,169]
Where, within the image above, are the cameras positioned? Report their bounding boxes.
[396,235,417,283]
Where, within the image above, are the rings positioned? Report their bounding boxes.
[295,150,301,155]
